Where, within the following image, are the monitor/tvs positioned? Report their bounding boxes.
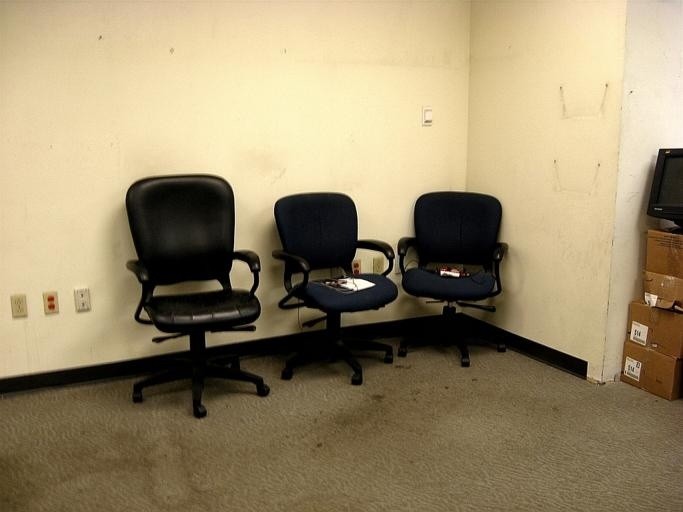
[646,147,683,234]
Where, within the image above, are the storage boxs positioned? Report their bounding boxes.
[645,227,683,279]
[621,340,683,400]
[641,270,682,313]
[627,299,682,360]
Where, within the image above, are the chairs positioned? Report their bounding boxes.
[122,173,269,417]
[272,191,398,386]
[395,192,508,368]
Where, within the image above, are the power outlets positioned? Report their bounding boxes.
[370,256,385,275]
[350,258,361,275]
[41,290,59,316]
[10,293,28,318]
